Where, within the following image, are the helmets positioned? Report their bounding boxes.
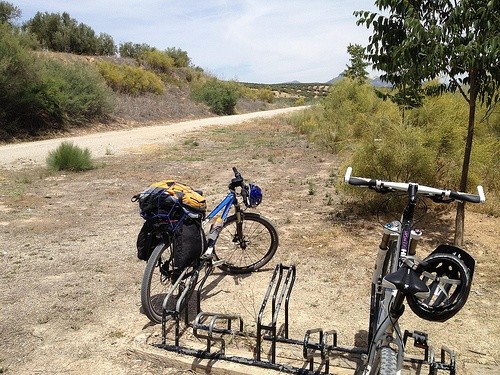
[244,183,262,208]
[403,244,476,322]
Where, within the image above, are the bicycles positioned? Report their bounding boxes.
[139,164,281,325]
[342,167,487,375]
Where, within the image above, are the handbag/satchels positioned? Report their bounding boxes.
[175,221,207,267]
[137,221,162,261]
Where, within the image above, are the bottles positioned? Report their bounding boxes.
[207,216,222,240]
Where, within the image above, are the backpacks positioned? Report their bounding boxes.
[139,179,206,221]
